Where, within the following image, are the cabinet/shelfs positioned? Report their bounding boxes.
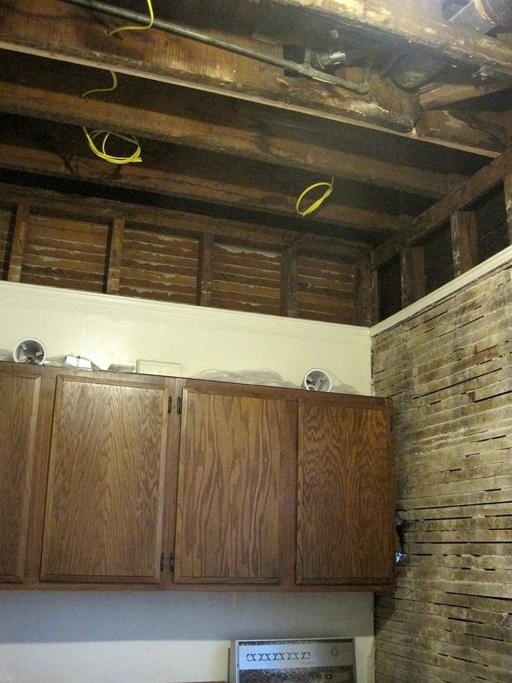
[0,372,171,585]
[172,387,396,586]
